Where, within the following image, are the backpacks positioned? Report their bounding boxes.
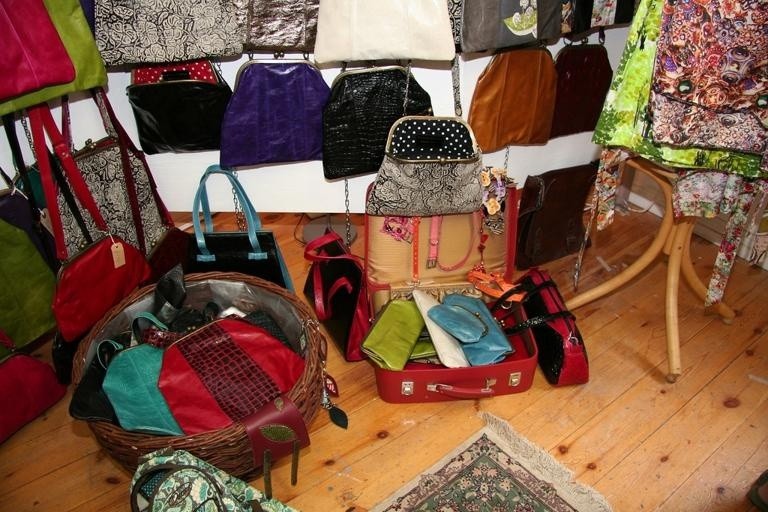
[124,447,295,511]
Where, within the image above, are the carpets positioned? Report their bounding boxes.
[367,411,613,511]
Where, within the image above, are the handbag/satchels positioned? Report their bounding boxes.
[218,52,330,171]
[361,290,515,372]
[515,157,601,270]
[1,330,67,446]
[364,55,483,217]
[71,274,302,434]
[302,227,371,363]
[182,165,295,293]
[468,44,556,155]
[491,267,590,388]
[324,60,433,180]
[127,58,233,156]
[551,28,614,139]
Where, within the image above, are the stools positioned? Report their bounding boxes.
[561,146,760,383]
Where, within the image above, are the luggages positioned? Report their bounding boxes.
[363,173,539,404]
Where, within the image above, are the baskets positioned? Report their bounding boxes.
[70,272,329,485]
